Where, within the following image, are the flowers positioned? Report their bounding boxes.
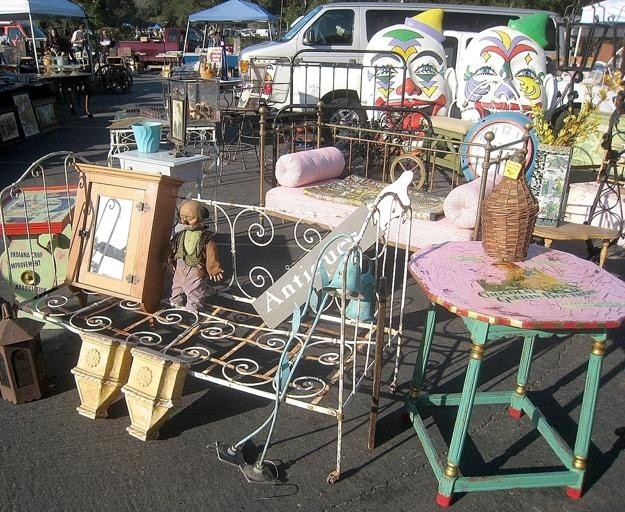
[533,82,604,147]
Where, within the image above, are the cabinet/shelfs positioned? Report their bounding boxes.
[116,146,211,200]
[65,162,184,330]
[167,76,223,128]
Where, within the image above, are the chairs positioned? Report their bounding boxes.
[202,61,274,183]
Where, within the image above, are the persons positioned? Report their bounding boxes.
[173,102,183,141]
[70,24,86,64]
[44,27,60,65]
[98,29,112,64]
[208,29,216,48]
[161,199,224,315]
[0,26,10,49]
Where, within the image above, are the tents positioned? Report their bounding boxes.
[182,0,280,66]
[0,0,94,75]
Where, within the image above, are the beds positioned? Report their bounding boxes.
[256,98,533,248]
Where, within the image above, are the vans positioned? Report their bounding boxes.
[239,2,581,147]
[0,23,47,57]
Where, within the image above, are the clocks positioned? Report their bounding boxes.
[458,110,540,182]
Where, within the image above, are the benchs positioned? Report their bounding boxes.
[0,151,412,486]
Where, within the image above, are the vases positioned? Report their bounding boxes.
[534,143,582,226]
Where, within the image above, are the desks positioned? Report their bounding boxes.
[406,238,625,505]
[107,118,140,169]
[533,223,621,269]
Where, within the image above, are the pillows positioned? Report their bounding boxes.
[444,173,504,229]
[275,146,346,187]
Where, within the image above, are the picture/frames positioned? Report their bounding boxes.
[0,185,79,236]
[170,95,189,149]
[13,93,42,140]
[35,98,61,128]
[1,111,21,144]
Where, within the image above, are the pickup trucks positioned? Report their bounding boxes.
[116,28,207,74]
